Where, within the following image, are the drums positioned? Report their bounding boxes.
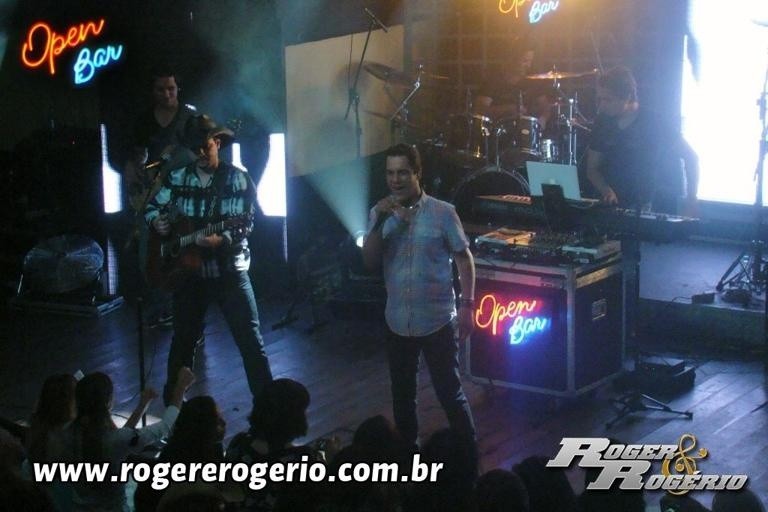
[452,166,530,226]
[438,113,492,164]
[495,116,541,168]
[539,138,559,163]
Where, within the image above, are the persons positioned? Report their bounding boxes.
[142,112,276,409]
[124,66,194,328]
[471,36,553,131]
[584,62,703,220]
[363,141,482,445]
[20,371,767,512]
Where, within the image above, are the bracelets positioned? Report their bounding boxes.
[459,298,474,310]
[372,221,384,233]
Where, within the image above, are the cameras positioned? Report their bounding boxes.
[316,439,330,450]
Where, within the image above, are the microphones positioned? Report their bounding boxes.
[370,194,396,236]
[365,8,388,33]
[144,151,170,170]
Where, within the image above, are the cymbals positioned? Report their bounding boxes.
[364,61,416,85]
[527,73,584,79]
[397,69,450,79]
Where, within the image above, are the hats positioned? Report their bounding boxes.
[179,112,235,152]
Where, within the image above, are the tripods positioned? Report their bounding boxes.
[716,67,768,296]
[606,241,693,429]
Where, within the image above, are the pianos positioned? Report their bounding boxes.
[471,194,698,242]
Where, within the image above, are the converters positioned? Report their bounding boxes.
[691,290,715,301]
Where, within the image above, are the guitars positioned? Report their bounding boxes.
[128,120,243,211]
[138,212,255,284]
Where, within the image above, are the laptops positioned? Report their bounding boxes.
[526,161,600,202]
